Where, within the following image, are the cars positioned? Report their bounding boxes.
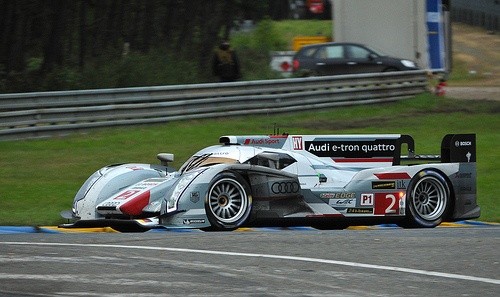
[292,42,420,77]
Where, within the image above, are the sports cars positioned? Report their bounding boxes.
[58,133,481,232]
